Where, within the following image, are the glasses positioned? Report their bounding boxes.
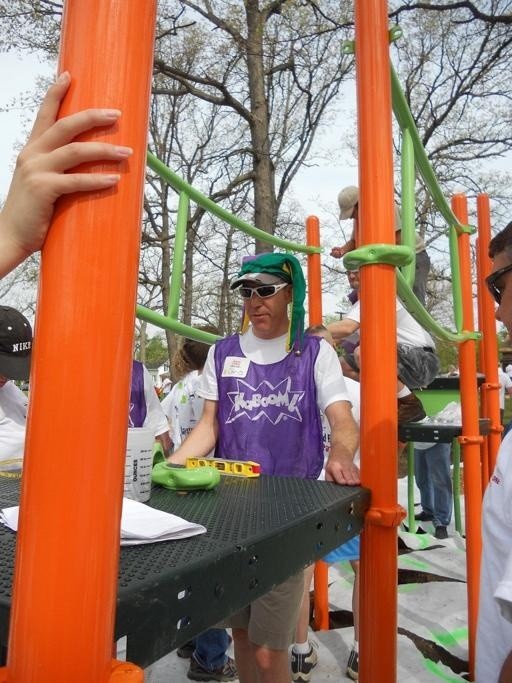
[239,283,288,299]
[486,263,512,304]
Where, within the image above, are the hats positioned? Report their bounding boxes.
[0,306,32,381]
[229,269,285,291]
[338,186,359,220]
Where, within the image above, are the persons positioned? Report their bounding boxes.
[0,303,33,463]
[0,72,133,282]
[413,443,453,539]
[291,324,360,682]
[161,374,172,398]
[161,253,360,683]
[506,363,512,379]
[330,184,430,308]
[160,325,236,681]
[326,254,439,424]
[498,361,512,426]
[474,221,511,683]
[124,359,173,465]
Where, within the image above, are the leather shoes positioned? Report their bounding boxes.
[414,511,433,521]
[398,393,426,424]
[435,526,448,539]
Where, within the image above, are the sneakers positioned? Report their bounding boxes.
[292,646,318,683]
[177,634,232,658]
[187,654,240,682]
[346,648,358,680]
